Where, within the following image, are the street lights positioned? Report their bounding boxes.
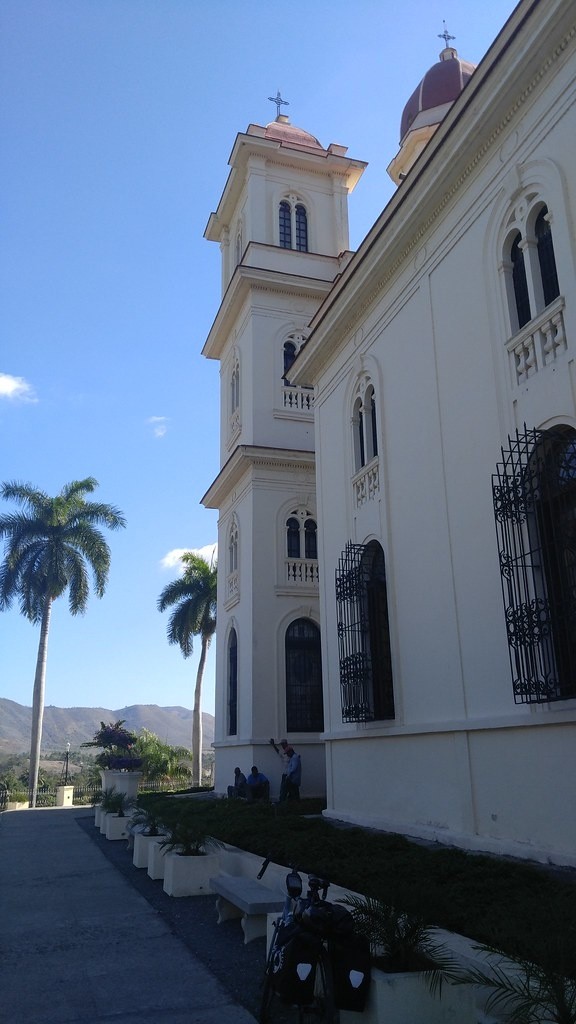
[64,742,71,787]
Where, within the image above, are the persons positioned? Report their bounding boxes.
[269,738,302,803]
[227,767,247,799]
[246,766,270,802]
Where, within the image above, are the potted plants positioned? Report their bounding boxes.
[80,720,224,898]
[316,877,576,1024]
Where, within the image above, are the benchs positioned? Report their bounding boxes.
[209,875,288,945]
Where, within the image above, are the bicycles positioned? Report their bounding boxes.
[254,852,344,1024]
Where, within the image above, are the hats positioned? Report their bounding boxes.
[278,739,287,744]
[284,745,292,755]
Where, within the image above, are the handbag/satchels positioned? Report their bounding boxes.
[272,897,370,1014]
